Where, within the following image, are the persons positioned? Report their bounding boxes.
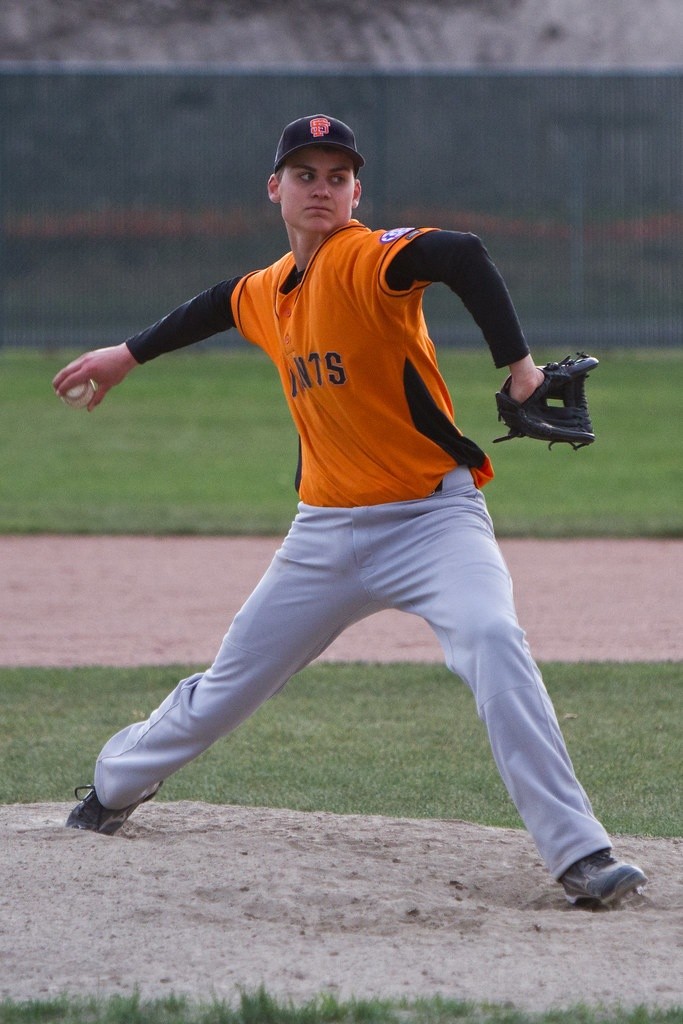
[54,114,647,908]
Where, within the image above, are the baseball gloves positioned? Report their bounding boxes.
[491,350,600,452]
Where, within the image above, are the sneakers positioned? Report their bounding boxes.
[63,778,166,834]
[560,850,647,909]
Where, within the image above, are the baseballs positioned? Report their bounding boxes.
[61,379,96,409]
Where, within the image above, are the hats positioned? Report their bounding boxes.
[275,114,365,179]
